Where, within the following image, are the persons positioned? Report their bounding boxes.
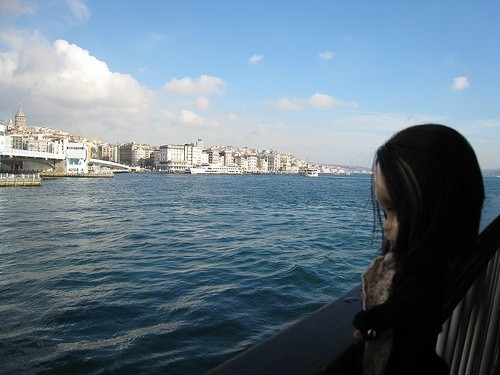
[350,125,486,375]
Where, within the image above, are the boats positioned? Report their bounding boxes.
[298,163,319,177]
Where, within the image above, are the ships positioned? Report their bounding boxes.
[189,163,243,175]
